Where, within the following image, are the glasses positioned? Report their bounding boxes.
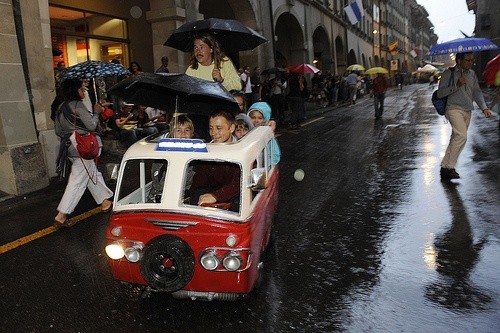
[463,58,475,63]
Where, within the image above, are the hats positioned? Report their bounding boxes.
[122,102,134,106]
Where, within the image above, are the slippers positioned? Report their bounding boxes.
[55,219,76,228]
[102,202,113,213]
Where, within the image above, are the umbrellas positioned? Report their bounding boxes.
[58,56,132,105]
[424,38,500,86]
[162,19,269,54]
[417,64,439,71]
[109,73,242,138]
[345,64,389,74]
[260,63,320,75]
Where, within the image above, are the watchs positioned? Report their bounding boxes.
[218,77,223,83]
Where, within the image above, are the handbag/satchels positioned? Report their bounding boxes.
[69,130,102,159]
[432,90,448,115]
[101,109,114,120]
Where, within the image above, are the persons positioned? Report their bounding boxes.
[50,77,114,225]
[188,109,241,214]
[394,72,404,89]
[147,114,196,203]
[227,90,280,169]
[54,60,169,149]
[428,72,435,90]
[156,56,170,73]
[372,73,389,117]
[186,34,242,91]
[437,53,491,177]
[237,66,371,122]
[487,71,500,115]
[128,62,144,74]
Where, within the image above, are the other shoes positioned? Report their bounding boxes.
[352,100,356,104]
[375,115,382,119]
[450,169,460,178]
[440,168,453,182]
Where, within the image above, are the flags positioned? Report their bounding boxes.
[389,41,398,70]
[409,46,419,57]
[343,0,364,25]
